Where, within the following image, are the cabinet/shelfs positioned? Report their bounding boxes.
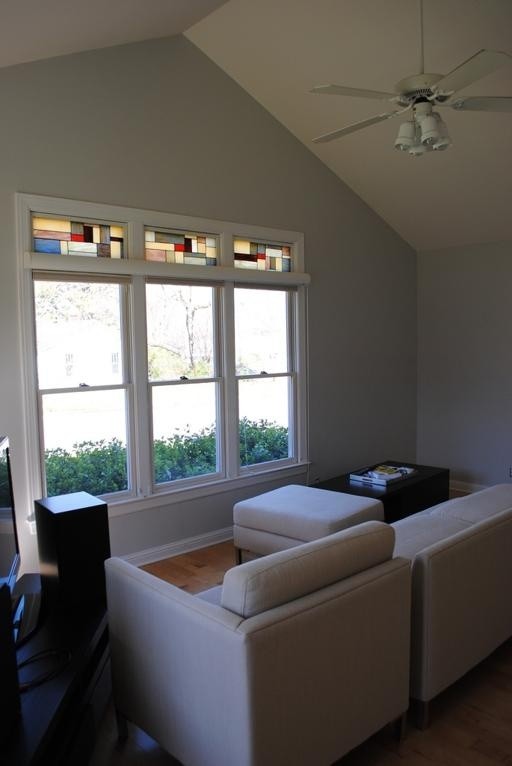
[0,571,117,765]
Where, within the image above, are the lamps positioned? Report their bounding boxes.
[392,100,452,159]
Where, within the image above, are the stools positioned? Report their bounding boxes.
[229,483,388,562]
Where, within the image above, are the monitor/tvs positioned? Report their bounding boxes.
[0,436,21,614]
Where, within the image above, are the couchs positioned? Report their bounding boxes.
[103,518,417,766]
[376,483,510,732]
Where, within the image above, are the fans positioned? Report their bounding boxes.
[308,0,512,144]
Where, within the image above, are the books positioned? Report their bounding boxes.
[348,463,418,486]
